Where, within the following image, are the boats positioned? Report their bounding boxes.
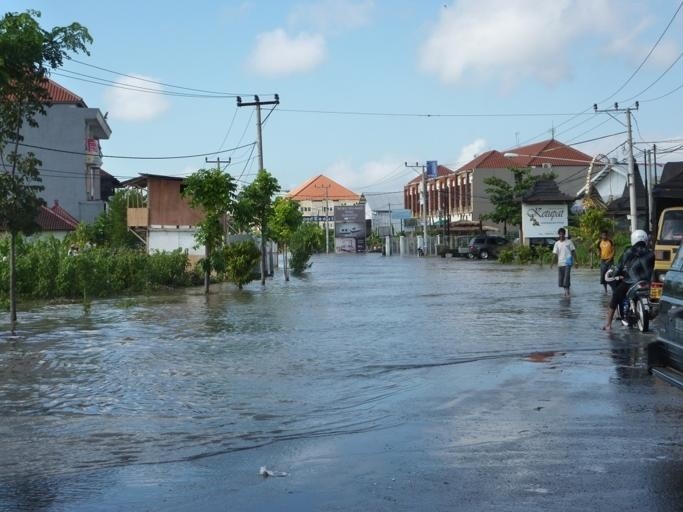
[349,226,361,233]
[338,227,351,234]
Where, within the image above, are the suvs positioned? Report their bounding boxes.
[643,239,683,391]
[611,265,653,333]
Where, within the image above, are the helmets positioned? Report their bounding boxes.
[631,230,648,246]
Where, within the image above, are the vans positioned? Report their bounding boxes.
[467,234,513,259]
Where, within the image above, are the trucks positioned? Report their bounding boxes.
[647,205,683,302]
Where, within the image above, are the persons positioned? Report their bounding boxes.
[595,227,615,295]
[600,228,659,332]
[549,225,579,302]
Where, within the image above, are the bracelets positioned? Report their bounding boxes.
[575,262,578,264]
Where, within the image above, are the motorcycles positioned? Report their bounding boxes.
[416,246,424,256]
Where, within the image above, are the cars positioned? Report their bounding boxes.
[373,243,382,252]
[511,237,560,257]
[340,210,356,220]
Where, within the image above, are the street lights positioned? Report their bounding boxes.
[501,152,638,234]
